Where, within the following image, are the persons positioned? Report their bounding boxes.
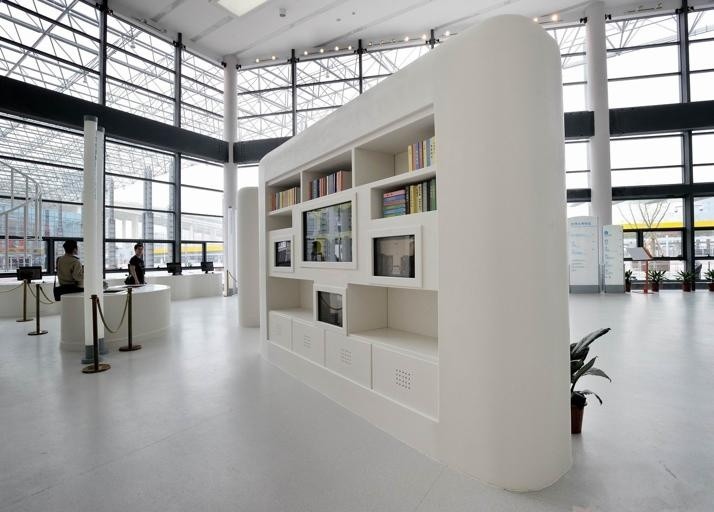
[54,238,83,295]
[126,242,146,285]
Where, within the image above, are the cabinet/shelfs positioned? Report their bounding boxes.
[262,101,438,424]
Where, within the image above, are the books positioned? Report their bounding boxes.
[379,133,437,219]
[270,169,349,211]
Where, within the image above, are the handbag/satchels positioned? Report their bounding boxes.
[53,257,78,302]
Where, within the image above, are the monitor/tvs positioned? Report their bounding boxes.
[19,266,42,283]
[167,263,182,275]
[201,262,214,274]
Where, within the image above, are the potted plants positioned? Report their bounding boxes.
[569,326,614,435]
[625,269,714,292]
